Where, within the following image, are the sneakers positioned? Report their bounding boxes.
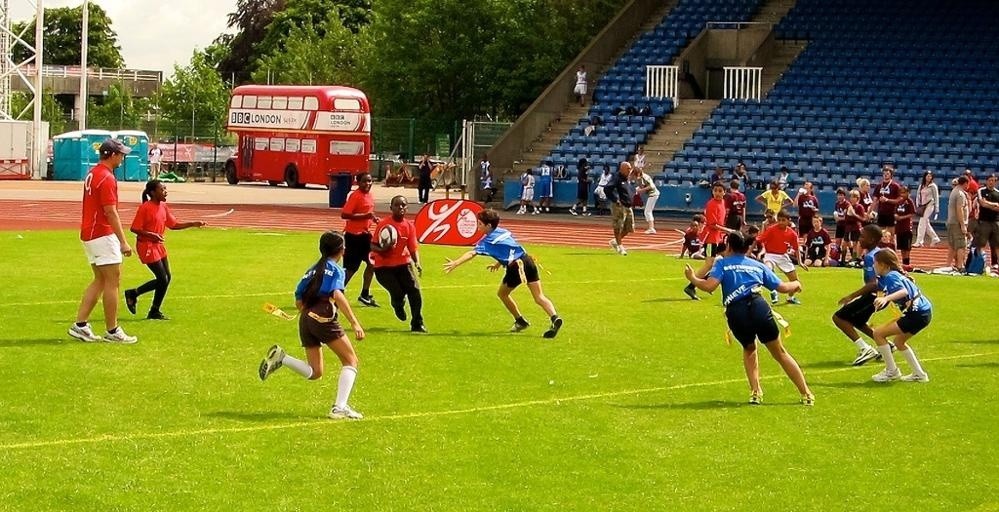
[258,344,288,381]
[391,303,407,322]
[124,287,140,315]
[146,308,170,322]
[798,391,816,407]
[103,325,139,346]
[327,402,364,421]
[515,207,658,257]
[850,340,930,384]
[358,293,381,309]
[410,322,429,334]
[749,389,765,405]
[66,322,103,344]
[542,317,564,338]
[509,319,530,332]
[768,291,802,306]
[911,238,941,249]
[683,286,702,302]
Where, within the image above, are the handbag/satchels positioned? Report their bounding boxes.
[915,203,927,218]
[965,246,987,276]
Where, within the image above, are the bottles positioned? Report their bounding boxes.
[798,188,808,195]
[833,211,838,220]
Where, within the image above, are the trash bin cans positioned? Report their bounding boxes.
[328,173,353,207]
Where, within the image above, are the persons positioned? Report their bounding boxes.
[679,163,999,304]
[602,159,634,257]
[516,160,568,217]
[442,210,562,342]
[677,231,818,407]
[573,65,589,107]
[871,246,936,385]
[148,143,163,181]
[369,195,432,332]
[412,155,437,208]
[258,230,363,419]
[832,222,902,366]
[340,171,385,312]
[473,156,499,205]
[125,180,208,320]
[67,138,137,344]
[575,156,662,236]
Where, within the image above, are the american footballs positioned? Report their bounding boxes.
[379,224,398,247]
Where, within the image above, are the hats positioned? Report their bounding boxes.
[96,138,134,157]
